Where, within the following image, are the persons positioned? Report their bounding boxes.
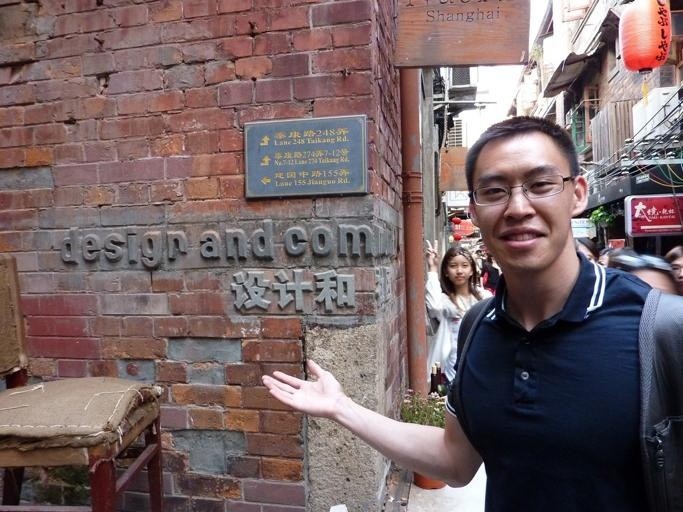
[258,115,682,512]
[468,234,683,314]
[423,238,494,396]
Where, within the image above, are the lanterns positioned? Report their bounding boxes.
[451,217,461,225]
[453,233,461,241]
[618,1,672,75]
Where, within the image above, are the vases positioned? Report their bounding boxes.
[414,472,446,489]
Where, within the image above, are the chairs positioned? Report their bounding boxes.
[1,255,161,511]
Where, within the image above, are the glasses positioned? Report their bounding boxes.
[467,173,575,208]
[611,254,671,272]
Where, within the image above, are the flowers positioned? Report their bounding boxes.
[400,389,446,428]
